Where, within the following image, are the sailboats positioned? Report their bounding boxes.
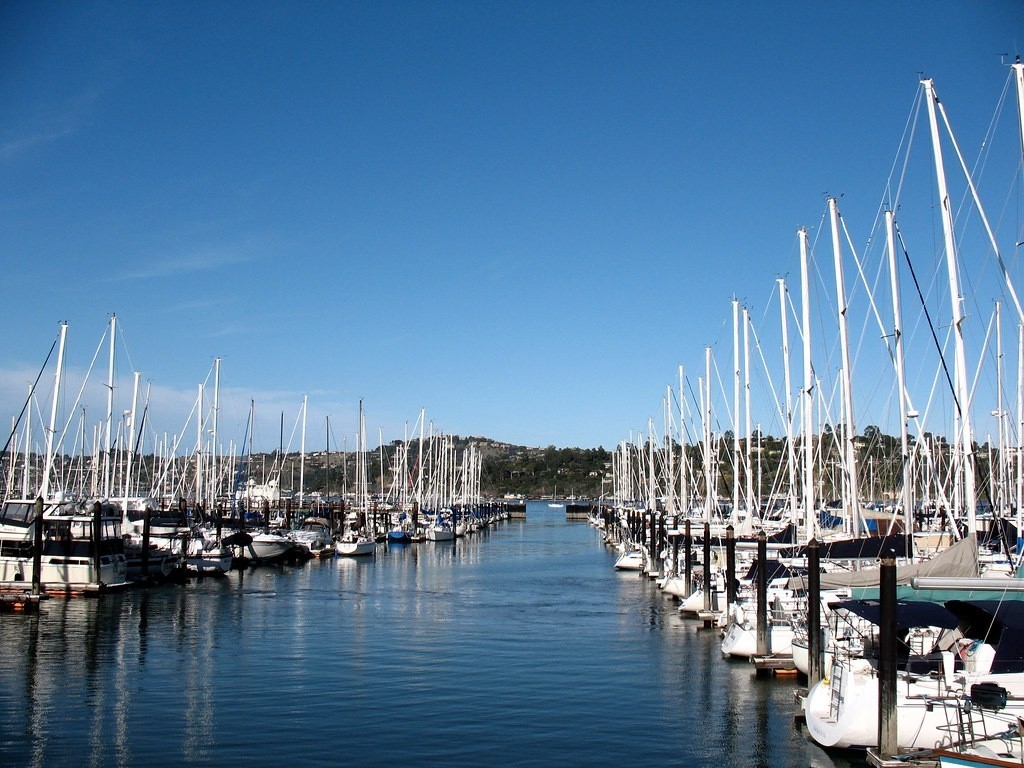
[0,310,510,601]
[585,50,1023,766]
[548,485,564,508]
[570,488,577,505]
